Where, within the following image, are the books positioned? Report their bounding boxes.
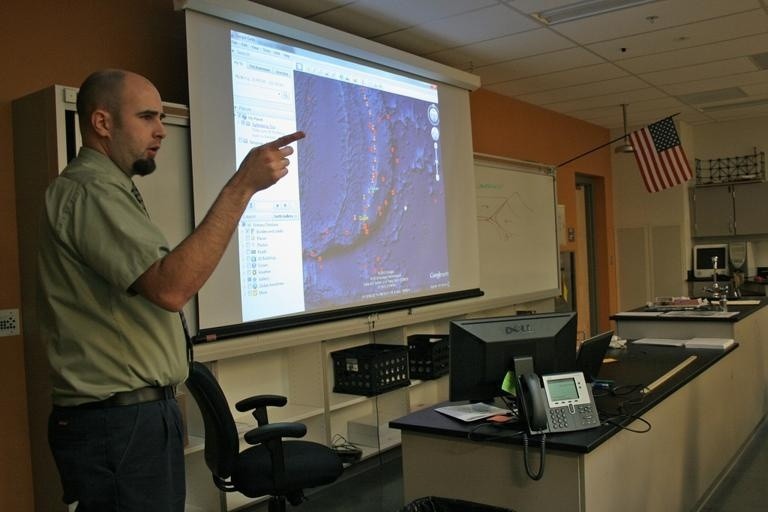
[685,336,734,352]
[434,400,517,424]
[631,336,687,347]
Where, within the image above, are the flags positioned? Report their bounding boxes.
[628,109,694,199]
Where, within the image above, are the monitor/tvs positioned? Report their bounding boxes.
[694,244,730,278]
[450,312,578,427]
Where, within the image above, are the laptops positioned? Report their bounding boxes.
[576,330,616,383]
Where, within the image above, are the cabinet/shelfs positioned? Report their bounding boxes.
[175,297,555,511]
[686,179,768,239]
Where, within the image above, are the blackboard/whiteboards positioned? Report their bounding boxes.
[73,113,562,351]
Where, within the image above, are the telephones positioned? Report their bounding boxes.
[516,370,601,436]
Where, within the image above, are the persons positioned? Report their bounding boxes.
[32,66,305,512]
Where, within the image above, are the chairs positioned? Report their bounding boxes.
[186,362,344,512]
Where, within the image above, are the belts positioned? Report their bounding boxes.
[50,385,180,412]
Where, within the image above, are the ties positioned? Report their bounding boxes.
[129,183,197,372]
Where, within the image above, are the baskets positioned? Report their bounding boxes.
[407,332,452,382]
[328,342,412,397]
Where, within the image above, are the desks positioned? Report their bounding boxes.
[389,295,766,511]
